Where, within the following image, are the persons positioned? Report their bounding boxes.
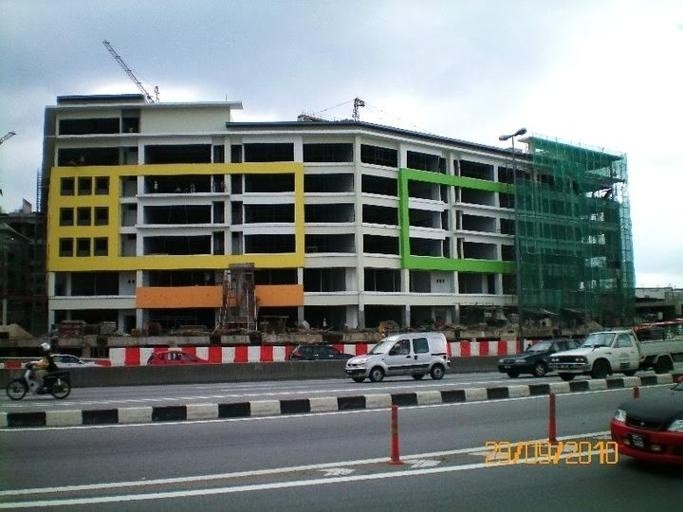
[26,342,58,394]
[49,324,59,351]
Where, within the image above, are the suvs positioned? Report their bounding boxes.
[288,344,354,359]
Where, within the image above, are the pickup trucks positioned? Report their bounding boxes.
[549,330,683,374]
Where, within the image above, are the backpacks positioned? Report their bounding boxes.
[47,363,58,372]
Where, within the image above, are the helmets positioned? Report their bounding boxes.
[40,343,51,351]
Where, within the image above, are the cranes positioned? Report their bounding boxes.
[297,98,367,122]
[102,38,159,104]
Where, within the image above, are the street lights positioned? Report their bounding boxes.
[500,128,527,339]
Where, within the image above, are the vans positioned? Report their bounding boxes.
[345,332,451,382]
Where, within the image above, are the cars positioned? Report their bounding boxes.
[497,338,582,377]
[147,351,211,363]
[610,377,682,465]
[40,353,105,367]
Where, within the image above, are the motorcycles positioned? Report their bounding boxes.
[6,361,71,401]
